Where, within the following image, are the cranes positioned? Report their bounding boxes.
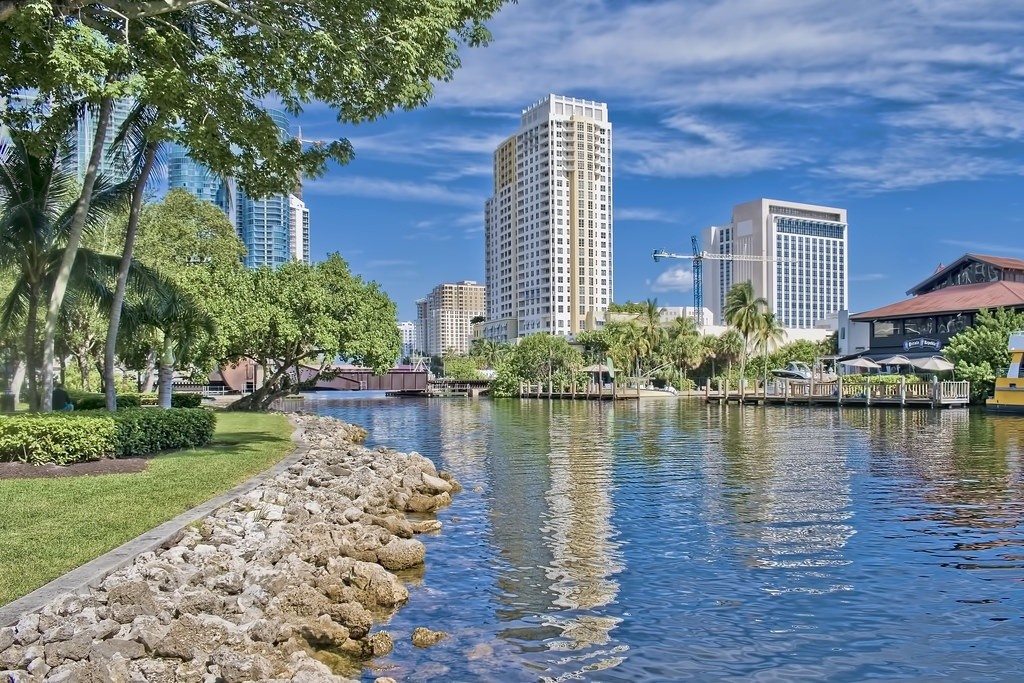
[652,237,800,327]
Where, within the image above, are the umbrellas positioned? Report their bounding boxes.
[578,364,624,372]
[910,355,956,381]
[875,356,915,374]
[840,357,881,376]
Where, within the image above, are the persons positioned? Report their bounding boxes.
[52,384,74,412]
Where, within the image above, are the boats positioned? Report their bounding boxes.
[985,331,1024,414]
